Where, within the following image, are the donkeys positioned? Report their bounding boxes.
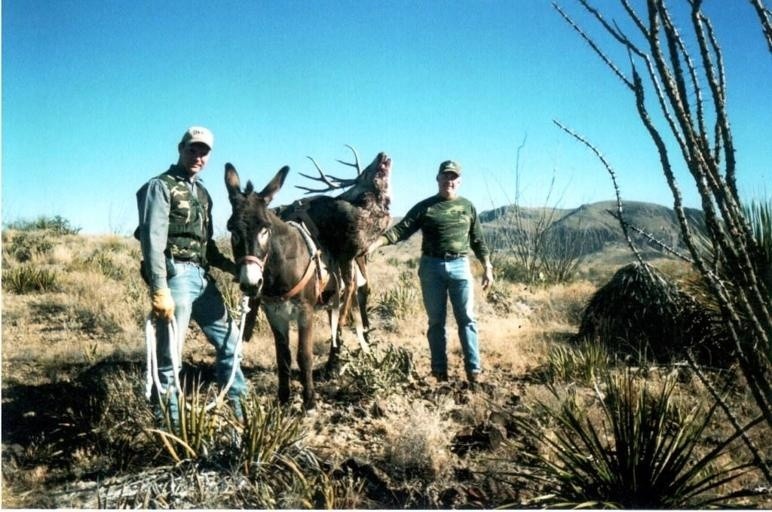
[221,161,368,411]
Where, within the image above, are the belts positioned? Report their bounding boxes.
[422,250,465,260]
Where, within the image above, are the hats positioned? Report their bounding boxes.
[439,160,462,177]
[182,126,213,151]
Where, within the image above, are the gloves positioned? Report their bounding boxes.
[150,288,174,324]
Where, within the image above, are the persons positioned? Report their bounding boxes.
[134,127,249,435]
[364,160,494,387]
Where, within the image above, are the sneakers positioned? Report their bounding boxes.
[468,373,484,393]
[418,371,449,387]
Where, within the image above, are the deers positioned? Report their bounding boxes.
[240,150,393,345]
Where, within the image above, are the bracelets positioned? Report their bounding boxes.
[483,265,493,269]
